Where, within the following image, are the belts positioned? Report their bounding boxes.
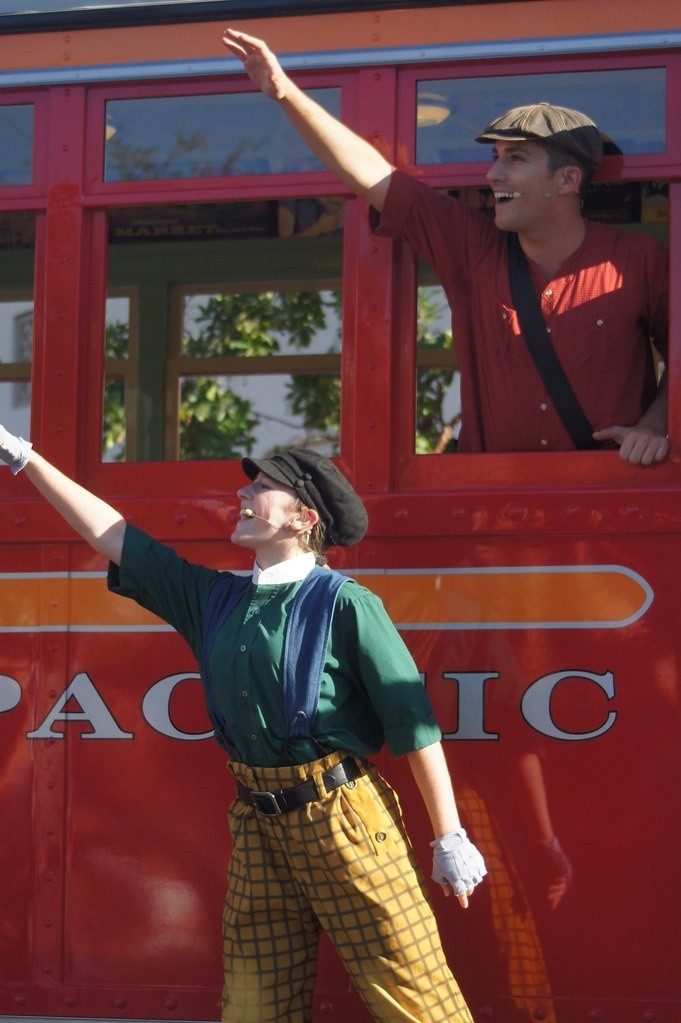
[236,757,370,817]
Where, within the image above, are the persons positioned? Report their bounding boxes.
[221,29,673,465]
[0,424,486,1023]
[381,589,573,1023]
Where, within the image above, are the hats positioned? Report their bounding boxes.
[242,447,368,547]
[476,97,603,167]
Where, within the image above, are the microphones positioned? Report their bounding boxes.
[245,509,278,530]
[512,192,553,198]
